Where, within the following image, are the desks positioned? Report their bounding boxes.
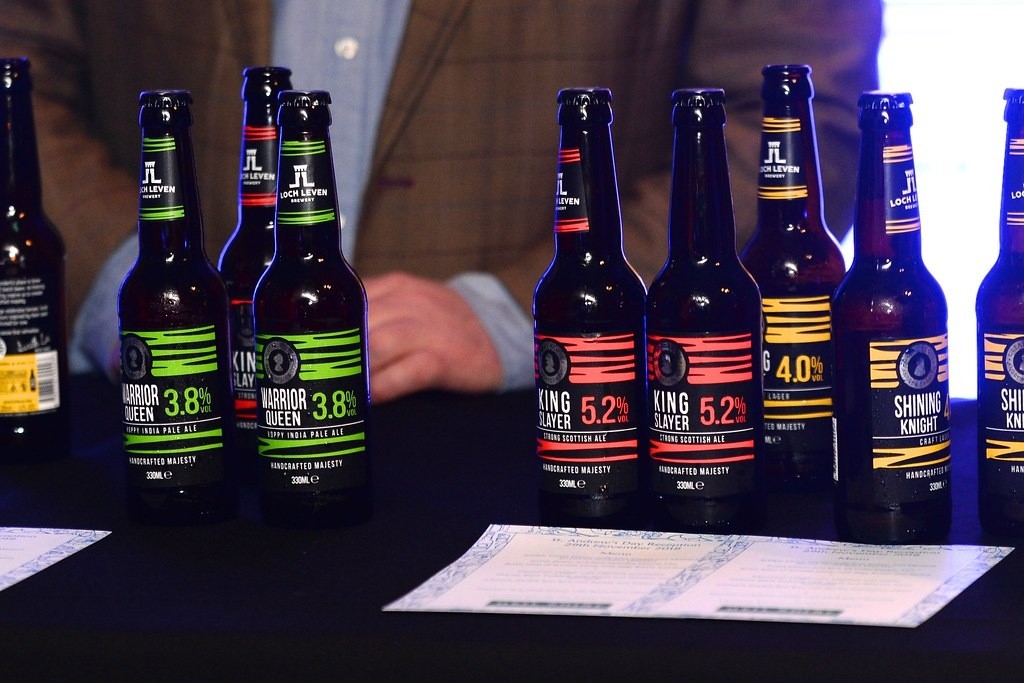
[0,380,1024,683]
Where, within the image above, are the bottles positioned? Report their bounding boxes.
[742,62,846,492]
[646,87,766,516]
[213,65,295,447]
[529,83,648,506]
[830,90,954,525]
[0,55,74,465]
[975,88,1024,508]
[116,87,235,512]
[252,91,370,510]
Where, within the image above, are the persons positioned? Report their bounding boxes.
[0,0,884,403]
